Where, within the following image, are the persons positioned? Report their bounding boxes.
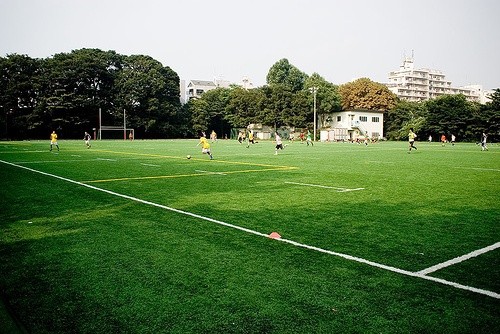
[238,130,258,148]
[289,132,294,143]
[408,128,417,153]
[441,135,445,146]
[372,135,380,143]
[197,133,213,160]
[210,130,217,143]
[306,129,314,146]
[481,133,488,151]
[84,132,91,149]
[129,132,133,140]
[355,131,361,145]
[428,135,431,143]
[50,131,59,152]
[300,132,304,144]
[452,134,455,146]
[365,131,368,146]
[274,131,288,155]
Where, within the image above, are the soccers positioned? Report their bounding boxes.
[187,155,191,159]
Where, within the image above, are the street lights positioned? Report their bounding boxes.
[309,86,320,141]
